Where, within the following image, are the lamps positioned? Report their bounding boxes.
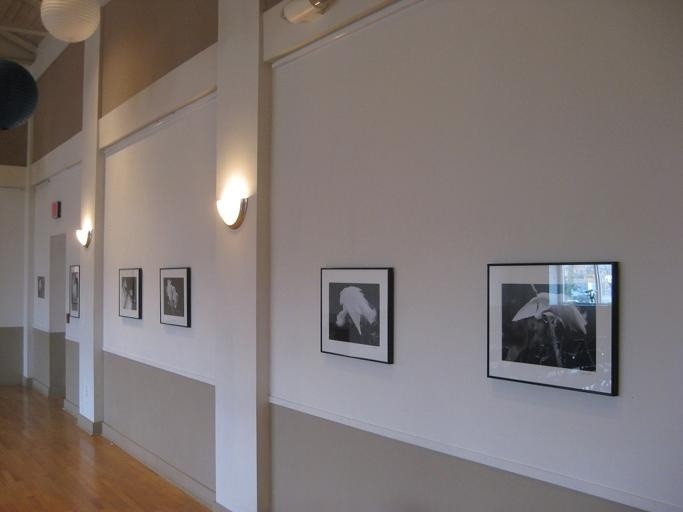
[0,57,38,130]
[75,228,92,247]
[279,1,327,24]
[214,197,246,228]
[40,0,101,44]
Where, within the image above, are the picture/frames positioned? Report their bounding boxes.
[118,268,141,320]
[484,261,619,396]
[36,274,46,300]
[318,265,393,364]
[67,264,80,318]
[158,266,190,328]
